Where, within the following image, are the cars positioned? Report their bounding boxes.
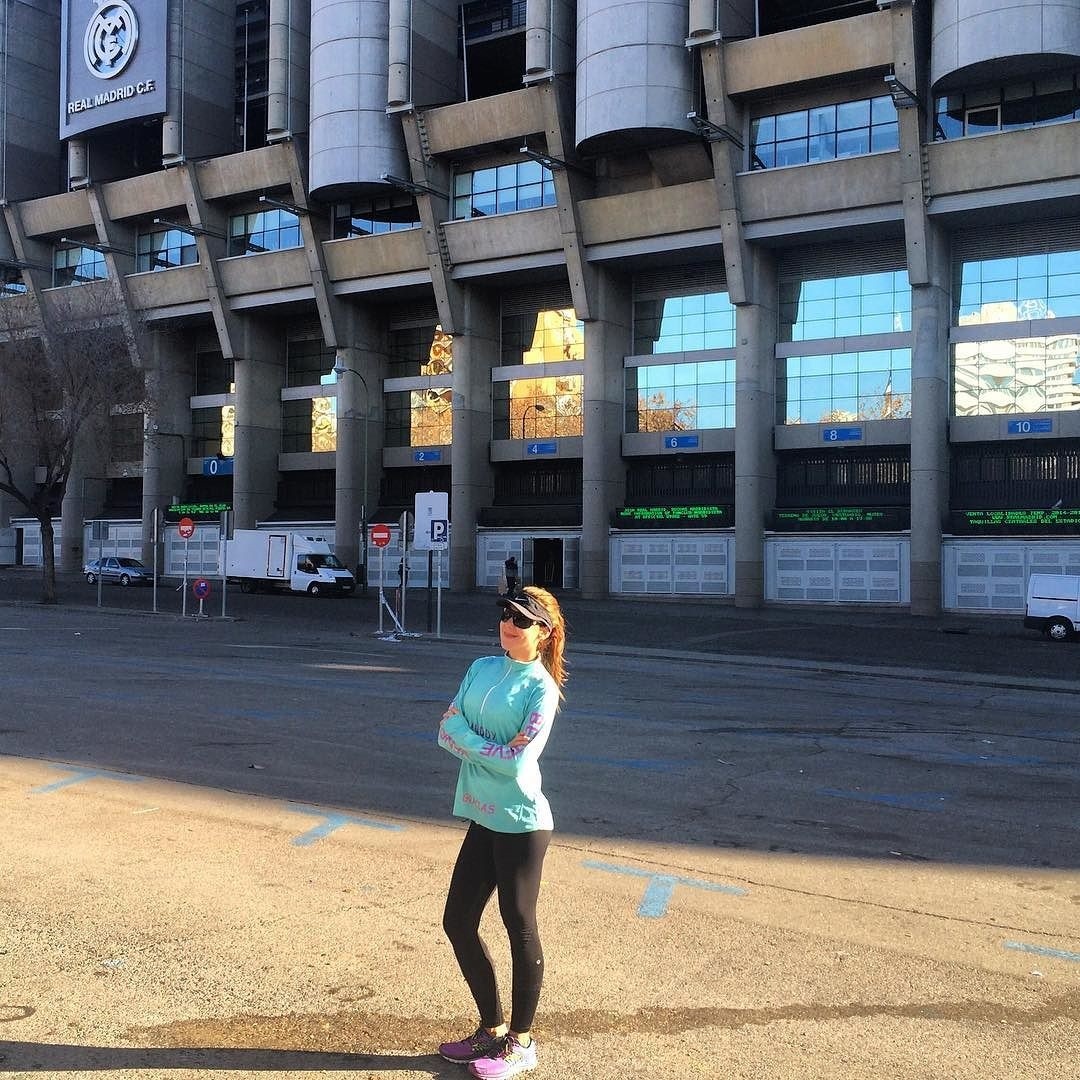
[83,556,162,588]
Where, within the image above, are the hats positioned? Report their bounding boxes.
[496,590,552,633]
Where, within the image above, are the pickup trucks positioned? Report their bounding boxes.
[1022,572,1080,642]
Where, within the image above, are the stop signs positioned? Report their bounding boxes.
[370,524,392,548]
[178,517,195,538]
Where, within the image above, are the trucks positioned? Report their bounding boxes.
[217,527,356,599]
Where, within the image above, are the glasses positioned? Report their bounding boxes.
[500,606,533,629]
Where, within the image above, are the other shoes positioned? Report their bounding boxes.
[398,588,402,590]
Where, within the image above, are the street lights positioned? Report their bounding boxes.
[332,366,370,600]
[522,403,546,442]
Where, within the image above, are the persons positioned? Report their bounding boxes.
[505,557,518,591]
[437,587,565,1080]
[398,558,410,588]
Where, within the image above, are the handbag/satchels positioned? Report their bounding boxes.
[398,569,402,575]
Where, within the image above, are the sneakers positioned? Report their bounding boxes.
[439,1028,504,1064]
[466,1033,537,1080]
